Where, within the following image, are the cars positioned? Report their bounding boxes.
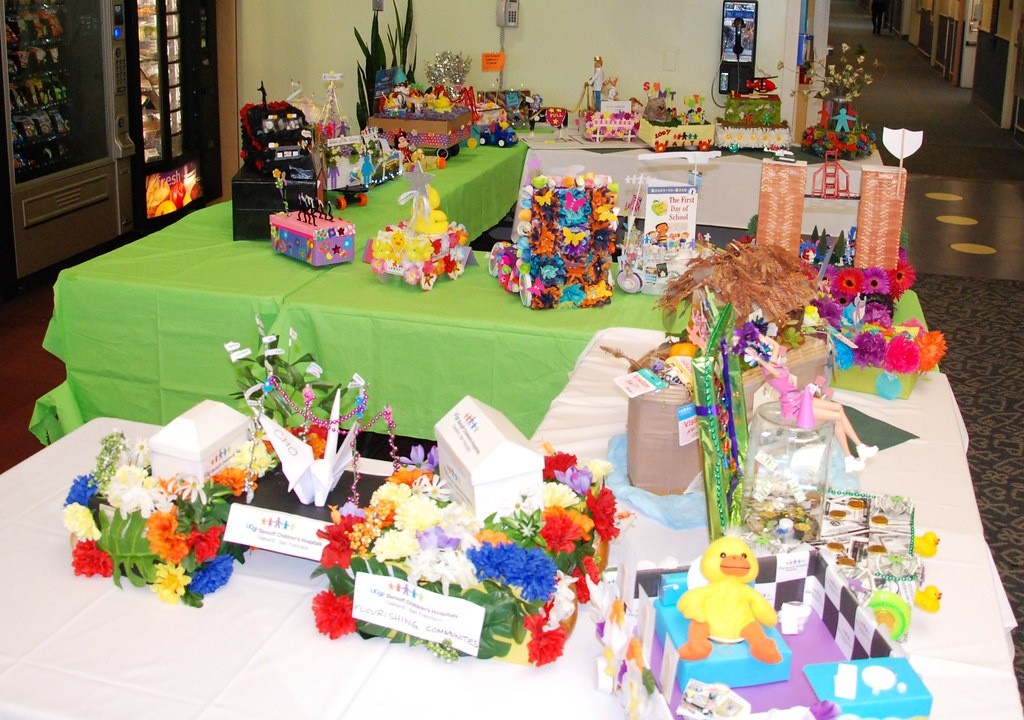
[478,120,519,147]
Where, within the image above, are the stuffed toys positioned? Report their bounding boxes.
[676,538,781,663]
[412,187,449,235]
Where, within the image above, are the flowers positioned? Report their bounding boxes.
[62,424,329,609]
[817,245,918,307]
[776,41,882,102]
[811,296,948,374]
[310,437,636,670]
[371,229,468,291]
[798,125,878,160]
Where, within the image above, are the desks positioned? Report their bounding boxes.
[510,123,884,244]
[28,126,554,448]
[236,250,940,441]
[338,326,1024,720]
[1,416,415,720]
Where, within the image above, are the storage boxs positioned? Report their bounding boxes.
[232,164,323,242]
[829,289,931,401]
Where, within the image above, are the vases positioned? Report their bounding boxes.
[828,97,850,131]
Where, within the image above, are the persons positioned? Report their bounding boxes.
[872,0,886,34]
[731,321,877,473]
[586,57,604,112]
